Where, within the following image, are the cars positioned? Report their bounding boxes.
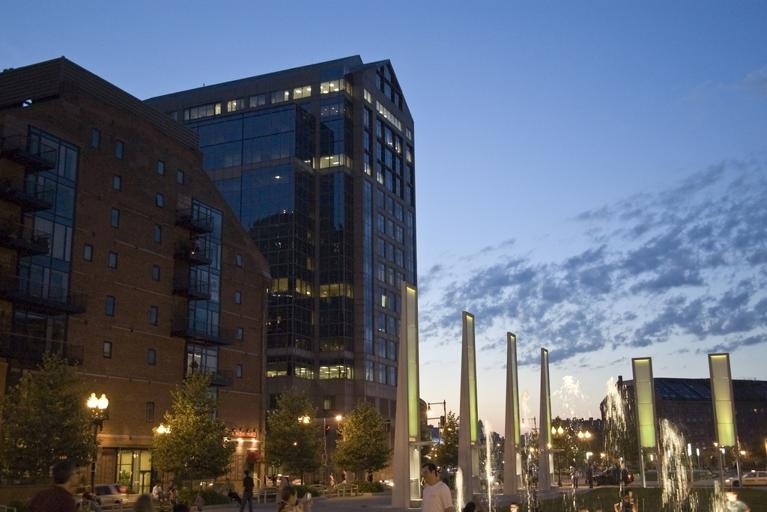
[446,464,503,488]
[656,460,710,482]
[266,470,306,489]
[724,469,767,487]
[73,483,131,509]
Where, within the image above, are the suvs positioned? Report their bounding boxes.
[591,466,634,488]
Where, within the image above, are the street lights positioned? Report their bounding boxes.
[551,424,565,490]
[296,413,311,482]
[577,426,593,485]
[322,414,344,460]
[86,391,110,495]
[426,400,447,443]
[157,423,173,500]
[520,415,540,451]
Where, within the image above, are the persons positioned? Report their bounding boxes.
[419,462,454,511]
[274,482,313,512]
[25,460,76,511]
[76,479,205,512]
[239,469,254,512]
[329,467,347,489]
[576,489,638,512]
[508,502,520,512]
